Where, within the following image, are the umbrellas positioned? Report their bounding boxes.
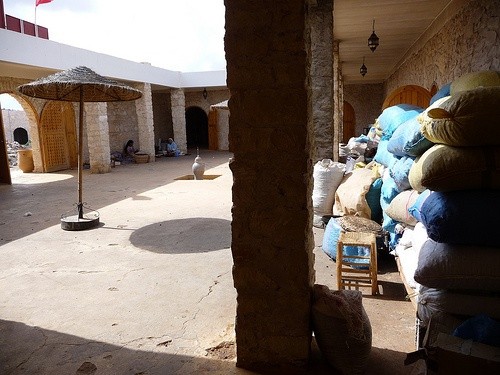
[36,0,53,6]
[17,65,143,220]
[211,100,229,110]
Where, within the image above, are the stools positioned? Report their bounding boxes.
[336,229,379,293]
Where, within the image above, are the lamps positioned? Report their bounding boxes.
[366,19,380,52]
[359,56,368,77]
[202,87,208,99]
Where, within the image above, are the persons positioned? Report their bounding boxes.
[124,140,140,155]
[166,138,177,150]
